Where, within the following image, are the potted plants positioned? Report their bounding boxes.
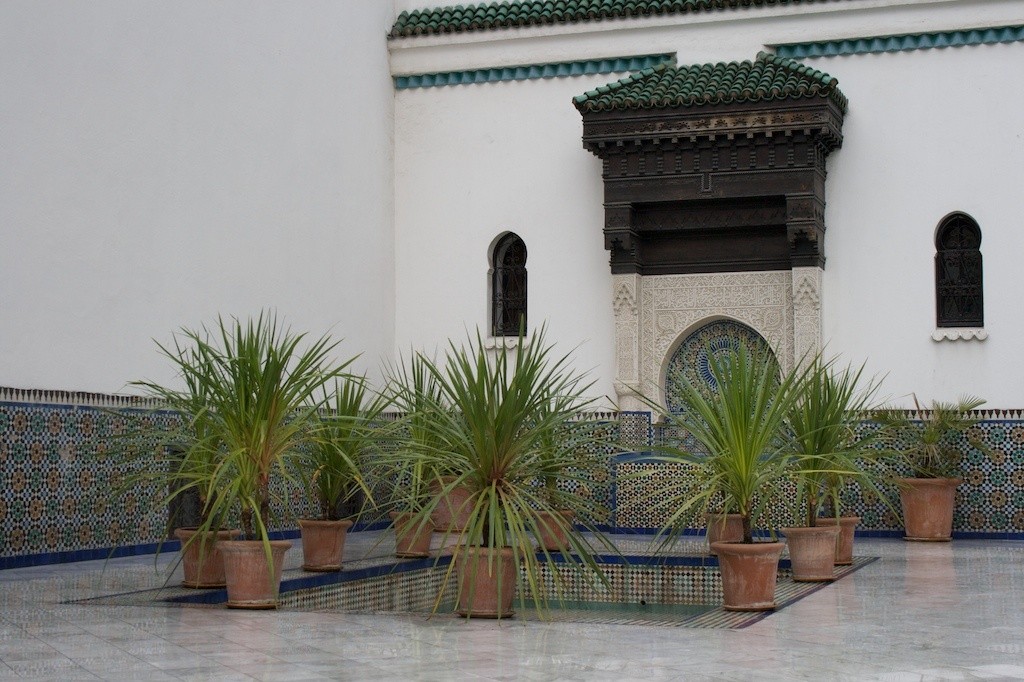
[72,307,992,627]
